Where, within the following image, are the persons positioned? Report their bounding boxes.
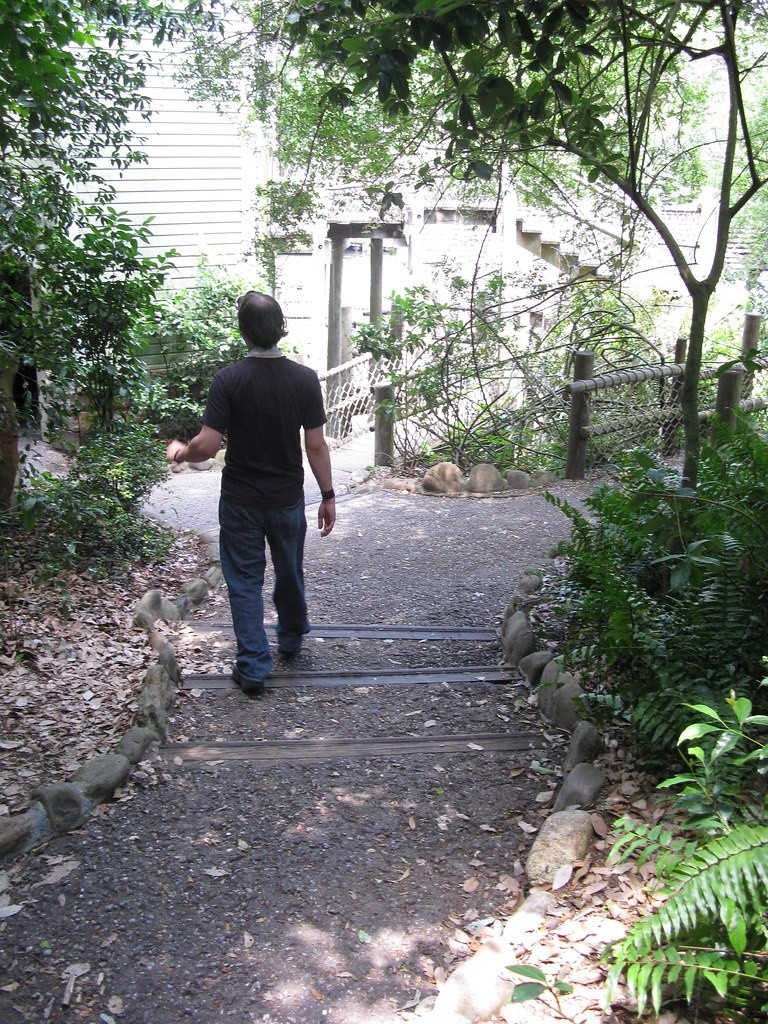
[164,290,337,692]
[11,349,39,429]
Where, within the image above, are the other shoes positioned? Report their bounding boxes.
[278,641,302,655]
[233,664,266,692]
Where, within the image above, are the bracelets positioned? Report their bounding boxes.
[321,489,335,501]
[173,450,184,464]
